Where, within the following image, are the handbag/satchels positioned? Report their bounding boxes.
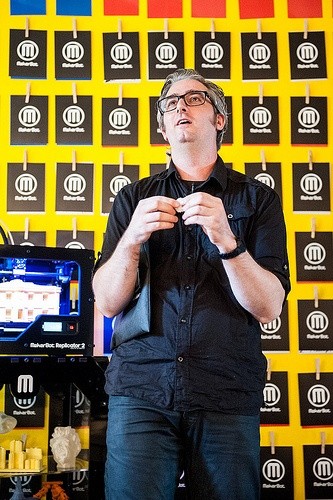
[106,237,152,350]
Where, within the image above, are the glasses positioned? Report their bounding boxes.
[152,90,219,115]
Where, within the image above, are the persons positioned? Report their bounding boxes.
[88,68,290,499]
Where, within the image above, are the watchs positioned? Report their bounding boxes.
[218,238,246,260]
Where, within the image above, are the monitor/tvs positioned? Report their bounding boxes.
[0,244,95,355]
